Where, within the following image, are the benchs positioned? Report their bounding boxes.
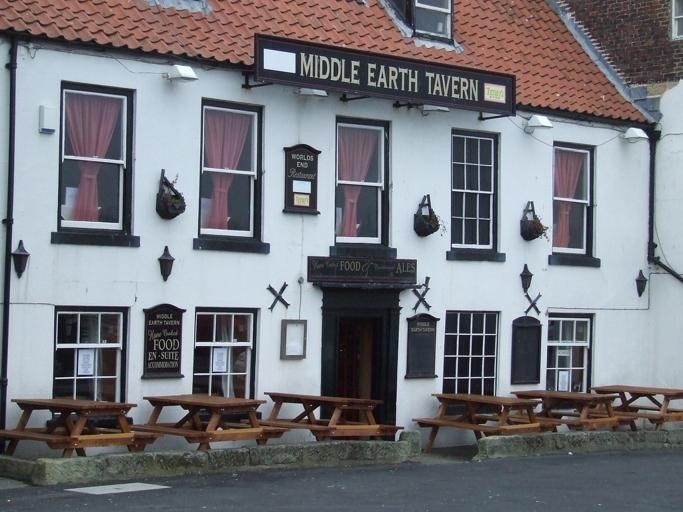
[410,385,683,454]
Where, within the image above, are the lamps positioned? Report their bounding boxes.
[515,113,552,128]
[392,100,450,117]
[162,64,199,81]
[291,87,327,98]
[618,127,649,141]
